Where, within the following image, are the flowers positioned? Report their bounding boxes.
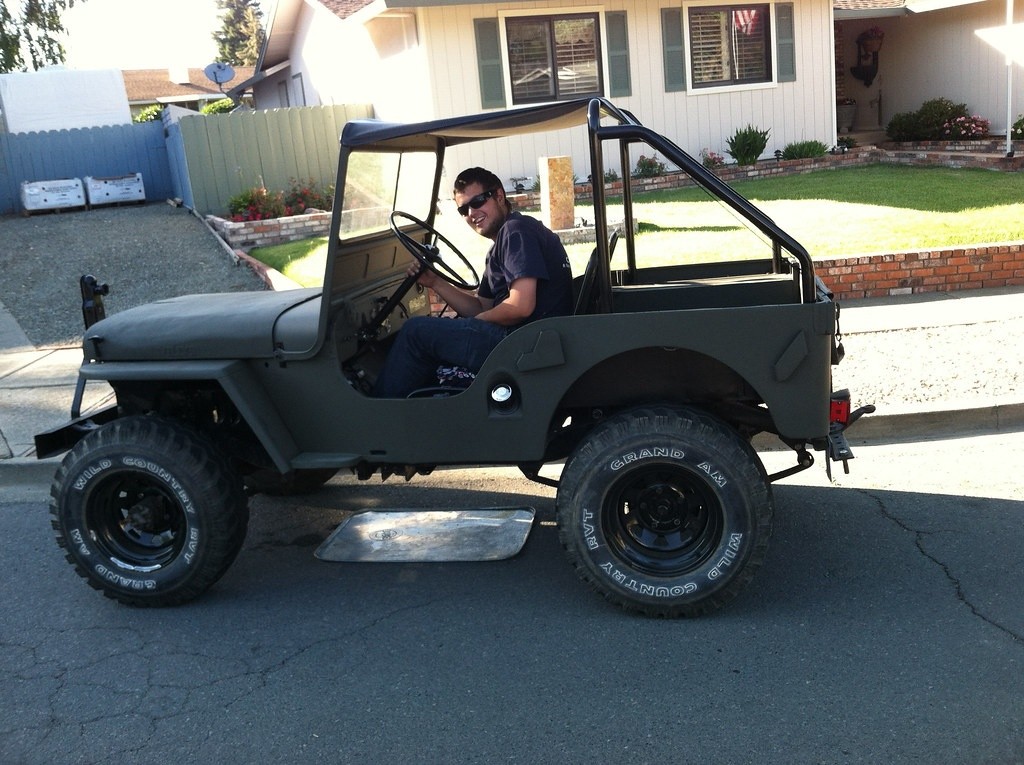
[861,26,883,40]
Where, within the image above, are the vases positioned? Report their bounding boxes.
[861,40,883,52]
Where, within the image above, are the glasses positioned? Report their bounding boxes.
[457,190,495,216]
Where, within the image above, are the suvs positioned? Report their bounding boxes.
[33,96,877,622]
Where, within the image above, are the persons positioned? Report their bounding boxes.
[370,167,576,399]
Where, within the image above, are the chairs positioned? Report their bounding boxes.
[570,225,622,317]
